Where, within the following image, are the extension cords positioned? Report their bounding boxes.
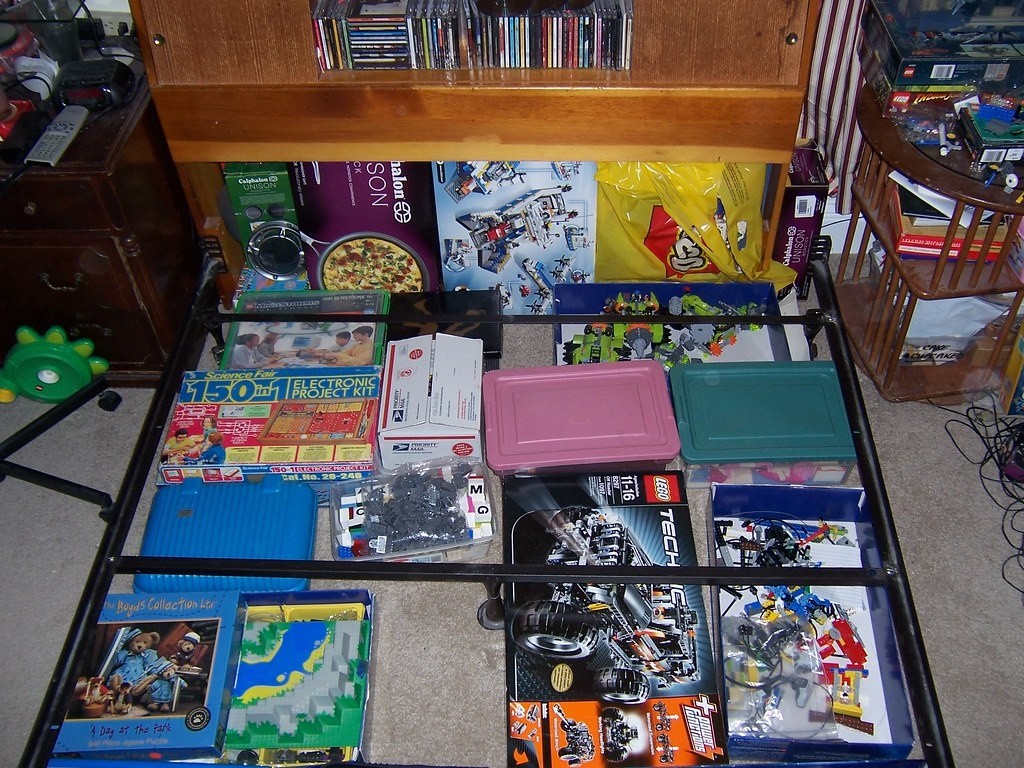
[1006,440,1024,482]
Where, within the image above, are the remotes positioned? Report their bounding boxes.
[24,105,88,168]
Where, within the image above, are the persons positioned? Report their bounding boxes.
[227,326,374,366]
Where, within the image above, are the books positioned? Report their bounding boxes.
[312,0,631,71]
[898,185,1006,228]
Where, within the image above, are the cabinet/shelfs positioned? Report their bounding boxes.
[834,82,1024,402]
[129,0,826,311]
[0,35,220,389]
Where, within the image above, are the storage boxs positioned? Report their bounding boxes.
[377,331,483,470]
[53,588,376,766]
[220,161,596,315]
[132,477,319,594]
[329,463,498,563]
[771,138,829,302]
[669,360,858,490]
[482,475,682,476]
[500,471,730,768]
[705,484,916,768]
[857,0,1024,167]
[158,365,386,483]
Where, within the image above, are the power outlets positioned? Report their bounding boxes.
[84,11,133,36]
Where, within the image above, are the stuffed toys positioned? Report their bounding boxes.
[80,626,200,715]
